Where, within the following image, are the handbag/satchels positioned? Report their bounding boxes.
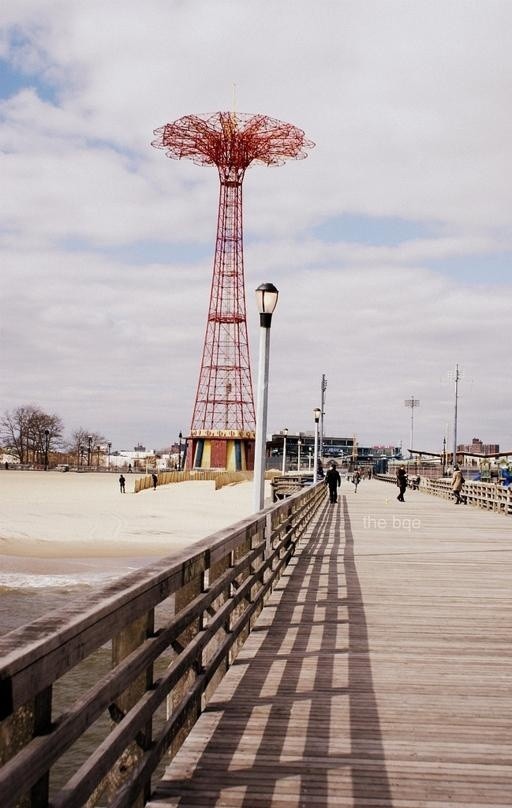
[460,477,465,483]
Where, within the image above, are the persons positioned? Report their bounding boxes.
[410,473,420,491]
[451,465,466,505]
[508,461,512,473]
[367,467,372,479]
[174,462,177,469]
[351,468,361,493]
[126,463,134,473]
[152,474,158,490]
[499,462,511,488]
[119,475,126,494]
[323,463,341,504]
[395,464,409,502]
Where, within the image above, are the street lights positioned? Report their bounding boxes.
[43,428,112,472]
[253,282,279,513]
[313,408,321,483]
[282,429,312,476]
